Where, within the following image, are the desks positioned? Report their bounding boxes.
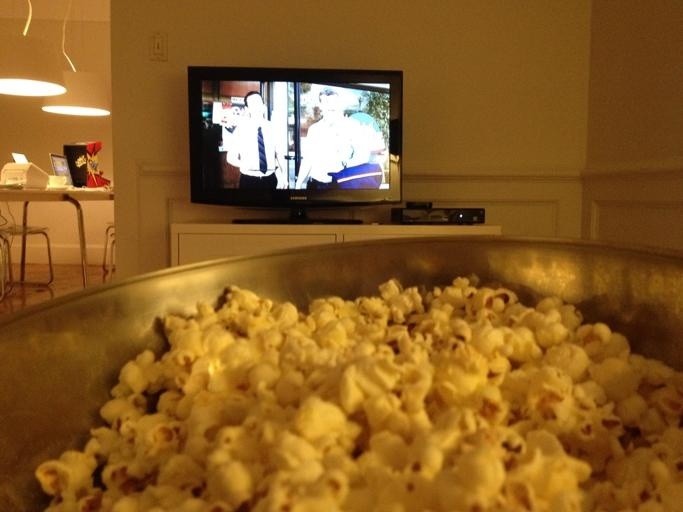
[0,187,115,301]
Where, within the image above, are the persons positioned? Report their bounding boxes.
[226,90,289,189]
[293,87,373,189]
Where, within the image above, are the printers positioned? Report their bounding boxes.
[1,162,48,190]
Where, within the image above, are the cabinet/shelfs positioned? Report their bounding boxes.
[170,222,502,268]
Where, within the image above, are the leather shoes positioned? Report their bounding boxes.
[257,127,267,174]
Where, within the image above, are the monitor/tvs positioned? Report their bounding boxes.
[188,65,404,225]
[49,153,74,185]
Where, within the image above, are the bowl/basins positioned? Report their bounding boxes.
[0,233,683,511]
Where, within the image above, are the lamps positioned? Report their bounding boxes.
[0,0,111,117]
